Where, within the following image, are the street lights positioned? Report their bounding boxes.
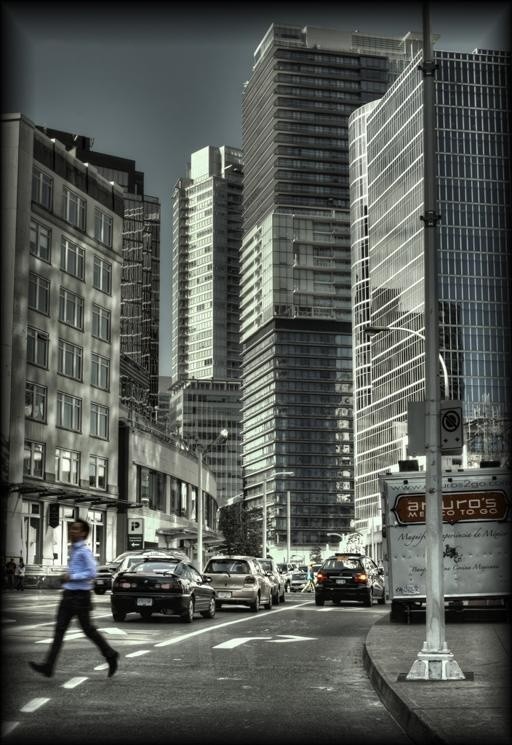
[196,428,228,575]
[261,471,296,557]
[365,324,453,468]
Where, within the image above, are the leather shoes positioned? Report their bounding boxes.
[106,651,119,677]
[29,661,53,677]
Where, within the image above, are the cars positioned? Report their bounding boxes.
[202,554,310,610]
[111,559,215,622]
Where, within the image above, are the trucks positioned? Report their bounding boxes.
[376,460,512,623]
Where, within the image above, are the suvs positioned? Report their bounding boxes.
[93,547,190,596]
[316,553,386,606]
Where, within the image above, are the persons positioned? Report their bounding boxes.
[5,558,16,589]
[28,517,120,678]
[300,564,316,594]
[16,558,25,591]
